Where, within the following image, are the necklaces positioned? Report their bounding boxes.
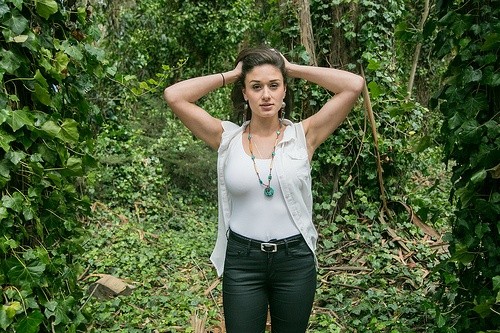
[248,120,282,196]
[252,136,276,159]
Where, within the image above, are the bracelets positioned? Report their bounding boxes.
[218,72,225,88]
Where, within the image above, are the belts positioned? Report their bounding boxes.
[229,231,304,252]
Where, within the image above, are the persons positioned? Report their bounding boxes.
[164,48,365,333]
